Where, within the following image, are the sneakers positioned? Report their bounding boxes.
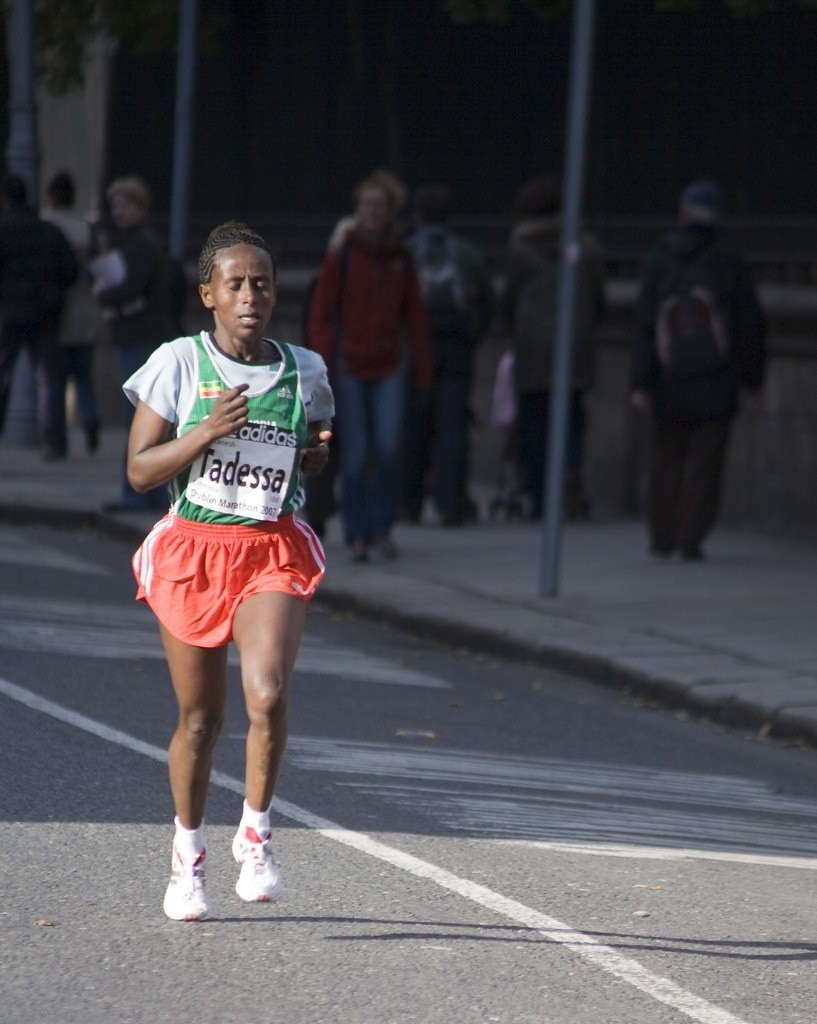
[230,820,281,903]
[164,843,212,920]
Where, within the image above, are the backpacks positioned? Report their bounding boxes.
[654,268,731,377]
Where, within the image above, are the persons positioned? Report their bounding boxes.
[0,172,80,439]
[36,172,101,463]
[303,170,479,563]
[122,221,335,921]
[91,178,187,516]
[497,178,606,520]
[627,181,769,564]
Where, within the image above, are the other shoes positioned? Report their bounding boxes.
[344,534,370,565]
[107,502,141,513]
[652,535,673,555]
[372,535,401,559]
[680,544,700,561]
